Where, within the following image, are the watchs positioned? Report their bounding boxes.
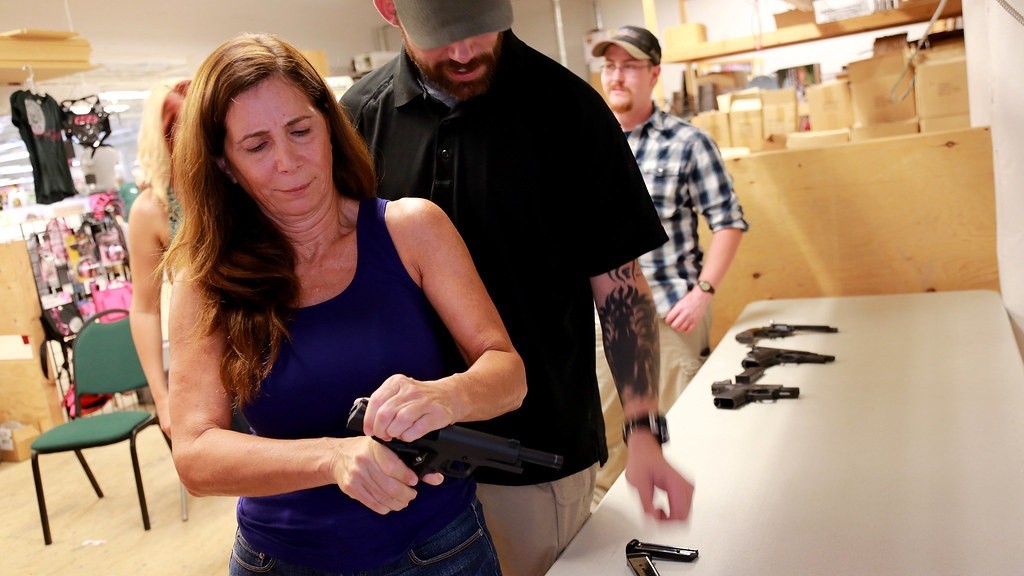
[696,281,715,296]
[621,412,669,445]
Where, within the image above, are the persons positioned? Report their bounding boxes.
[339,0,696,575]
[574,27,747,511]
[128,81,255,439]
[167,39,507,576]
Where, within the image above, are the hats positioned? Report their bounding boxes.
[591,26,662,65]
[392,0,514,50]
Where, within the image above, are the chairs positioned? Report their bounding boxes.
[29,307,172,547]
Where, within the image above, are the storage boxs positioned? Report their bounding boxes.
[663,0,970,151]
[0,425,40,462]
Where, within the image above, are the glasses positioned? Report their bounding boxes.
[599,63,651,76]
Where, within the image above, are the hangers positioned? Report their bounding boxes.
[20,65,47,98]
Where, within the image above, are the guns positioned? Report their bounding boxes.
[741,346,835,369]
[344,399,565,483]
[711,379,800,410]
[735,323,837,343]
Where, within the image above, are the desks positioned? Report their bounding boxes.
[544,290,1024,576]
